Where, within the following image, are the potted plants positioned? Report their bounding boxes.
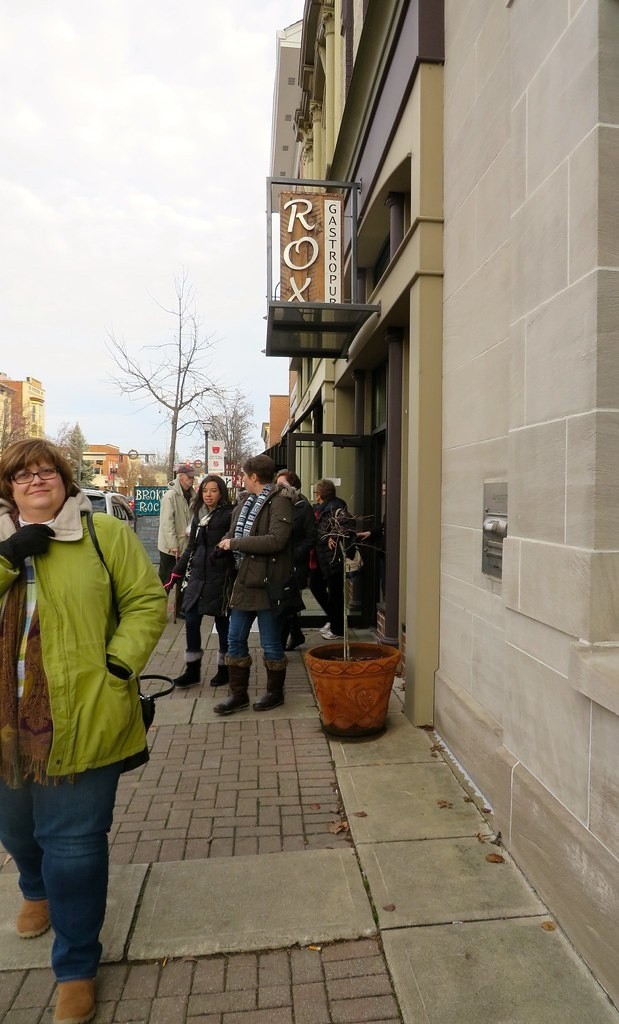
[303,499,400,739]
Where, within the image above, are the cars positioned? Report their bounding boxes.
[81,489,136,530]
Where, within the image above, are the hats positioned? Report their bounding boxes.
[178,465,194,477]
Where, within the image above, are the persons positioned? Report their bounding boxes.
[0,438,168,1024]
[157,453,388,714]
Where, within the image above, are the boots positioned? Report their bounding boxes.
[173,649,204,688]
[213,653,253,714]
[210,650,229,686]
[253,654,287,711]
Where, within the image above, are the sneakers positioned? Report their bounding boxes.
[16,898,51,939]
[53,980,96,1024]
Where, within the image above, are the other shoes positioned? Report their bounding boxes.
[322,631,344,640]
[319,623,331,633]
[285,634,305,651]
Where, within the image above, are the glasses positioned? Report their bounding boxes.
[10,468,59,484]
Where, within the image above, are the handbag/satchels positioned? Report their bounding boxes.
[264,575,306,619]
[135,674,176,735]
[345,550,364,578]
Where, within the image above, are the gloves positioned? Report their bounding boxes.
[107,662,130,680]
[0,524,55,566]
